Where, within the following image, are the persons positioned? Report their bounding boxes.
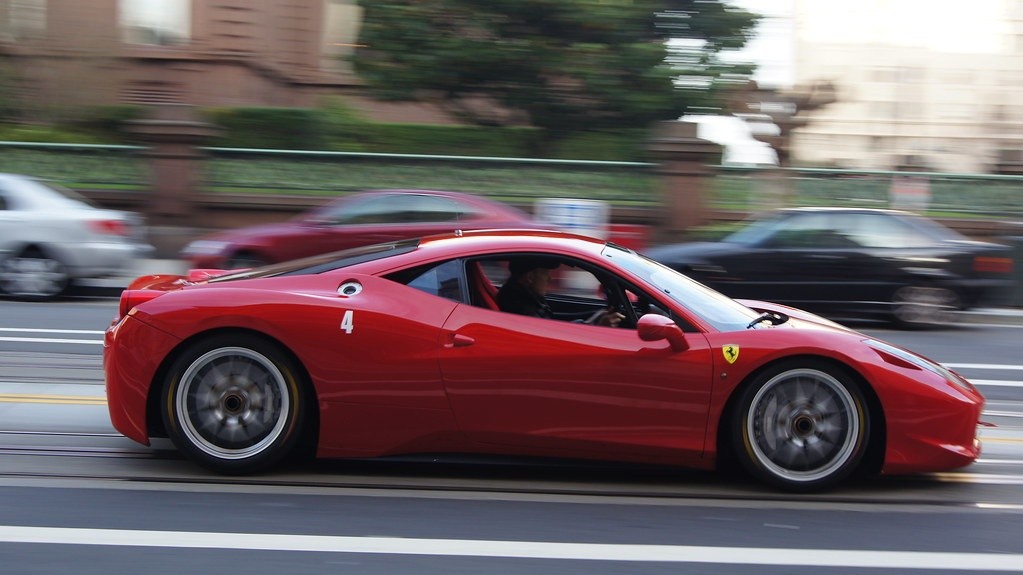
[496,258,626,329]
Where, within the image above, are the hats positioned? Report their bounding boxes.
[508,256,560,276]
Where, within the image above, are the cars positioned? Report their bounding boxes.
[182,187,560,271]
[0,173,159,301]
[639,205,1017,329]
[103,227,984,492]
